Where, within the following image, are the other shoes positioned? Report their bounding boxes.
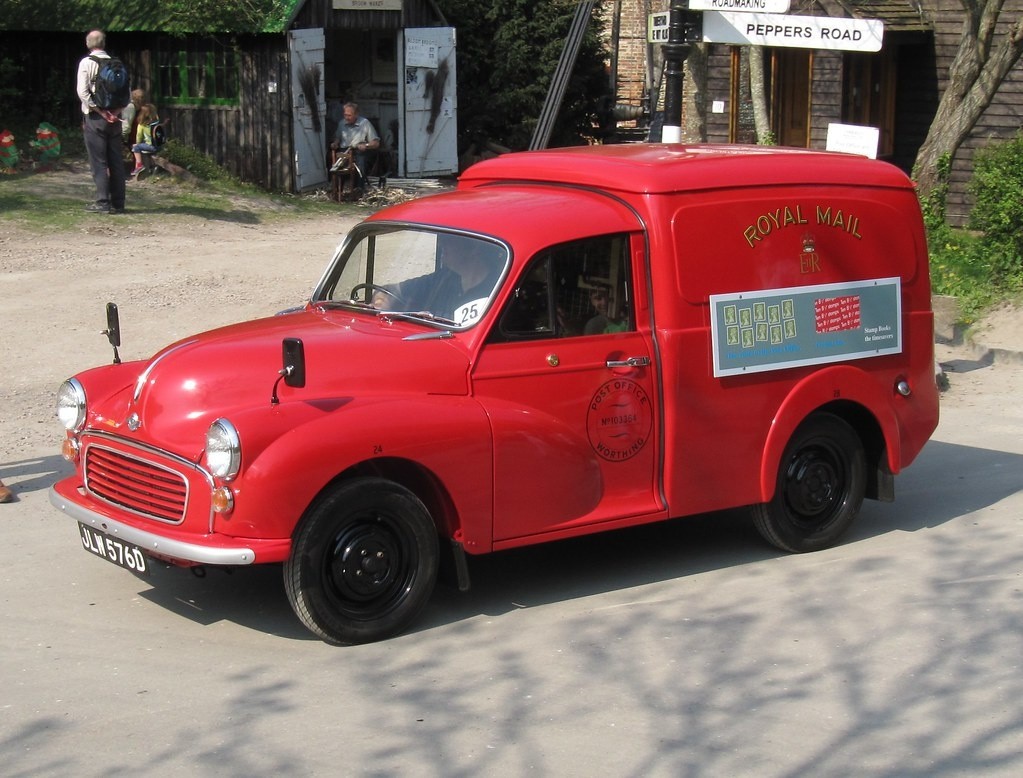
[353,187,363,201]
[130,164,146,175]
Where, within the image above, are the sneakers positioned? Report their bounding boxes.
[83,201,110,213]
[108,202,125,213]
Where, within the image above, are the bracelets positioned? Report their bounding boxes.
[365,143,368,148]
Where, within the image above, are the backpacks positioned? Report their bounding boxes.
[86,55,131,109]
[146,120,167,147]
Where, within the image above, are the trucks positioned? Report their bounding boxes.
[50,143,940,647]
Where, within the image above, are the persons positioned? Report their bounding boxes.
[77,30,125,213]
[122,89,147,147]
[330,102,381,190]
[372,234,537,329]
[582,286,628,335]
[130,104,158,175]
